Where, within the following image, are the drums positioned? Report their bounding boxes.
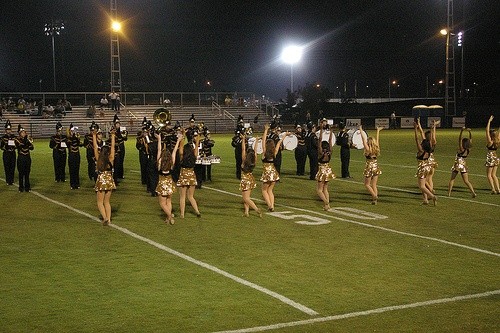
[315,129,337,147]
[202,157,212,165]
[209,155,221,164]
[279,131,299,151]
[248,137,263,155]
[195,157,202,164]
[347,128,368,149]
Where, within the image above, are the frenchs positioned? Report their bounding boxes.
[153,108,172,134]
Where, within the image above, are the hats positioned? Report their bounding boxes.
[307,120,315,129]
[136,113,211,137]
[5,119,12,130]
[269,122,282,129]
[56,121,64,131]
[68,120,103,137]
[338,121,345,129]
[320,116,327,122]
[113,114,121,125]
[293,121,301,129]
[16,124,26,135]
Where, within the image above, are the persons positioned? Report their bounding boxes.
[0,96,72,120]
[411,114,440,206]
[232,113,357,179]
[176,127,201,219]
[237,133,263,218]
[357,125,384,205]
[49,120,84,189]
[153,133,184,224]
[486,115,500,196]
[390,111,397,129]
[164,98,173,108]
[259,123,293,210]
[223,95,234,106]
[315,122,336,210]
[86,90,215,197]
[91,128,116,225]
[14,124,34,192]
[335,122,357,178]
[447,127,478,198]
[0,119,18,186]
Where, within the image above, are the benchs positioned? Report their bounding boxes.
[0,105,277,136]
